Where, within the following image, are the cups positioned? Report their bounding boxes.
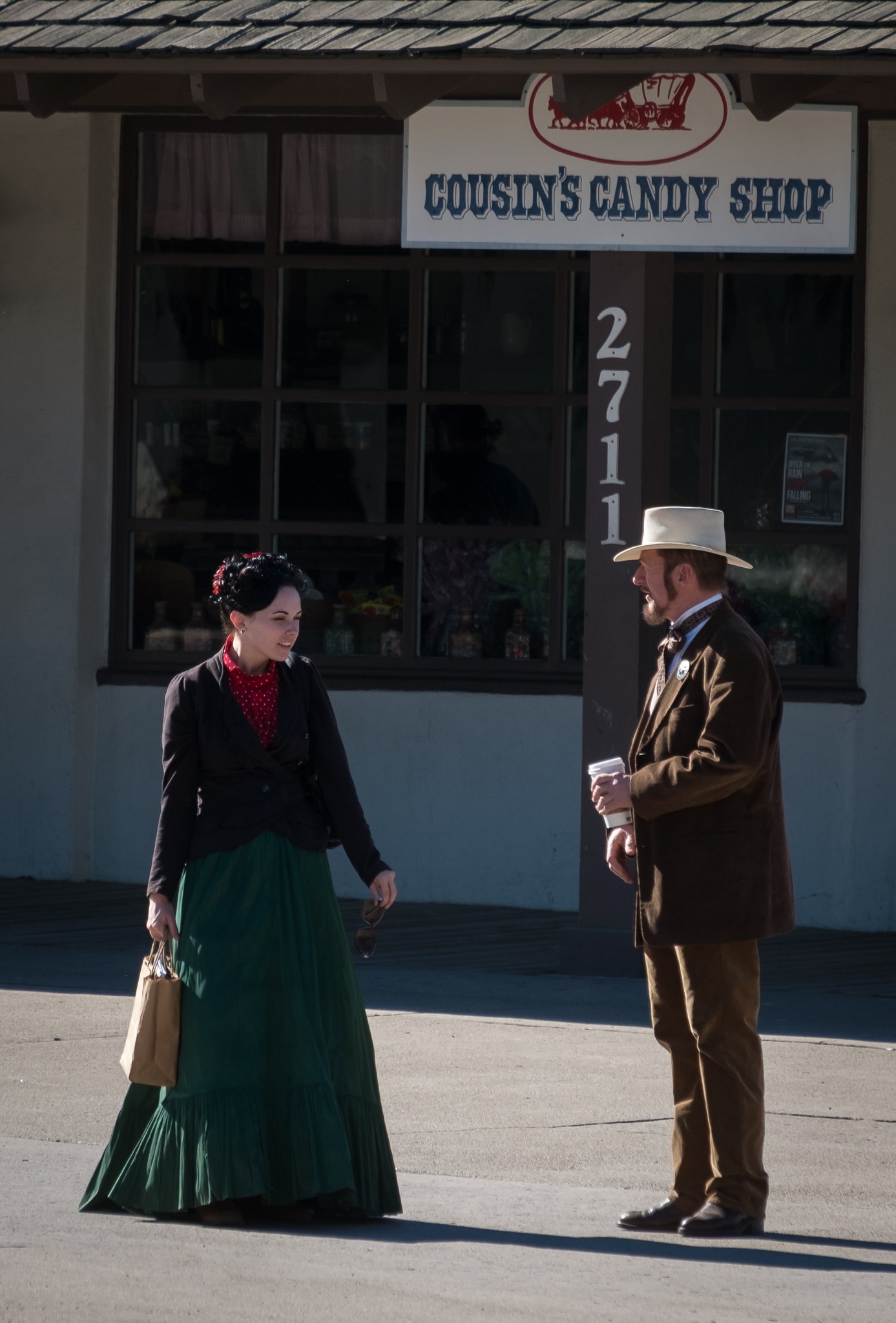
[588,757,632,829]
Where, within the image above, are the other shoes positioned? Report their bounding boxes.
[279,1205,317,1226]
[198,1202,241,1224]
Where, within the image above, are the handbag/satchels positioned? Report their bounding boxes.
[119,927,181,1086]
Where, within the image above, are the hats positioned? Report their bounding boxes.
[611,504,754,570]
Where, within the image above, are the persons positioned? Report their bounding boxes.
[78,551,411,1218]
[590,506,799,1237]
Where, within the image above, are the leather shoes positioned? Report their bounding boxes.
[619,1196,690,1231]
[677,1198,766,1238]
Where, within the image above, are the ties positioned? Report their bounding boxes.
[655,599,727,700]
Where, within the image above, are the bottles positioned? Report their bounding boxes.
[325,604,358,656]
[771,619,796,665]
[505,609,532,660]
[380,605,402,656]
[145,601,179,651]
[180,601,213,652]
[450,607,482,659]
